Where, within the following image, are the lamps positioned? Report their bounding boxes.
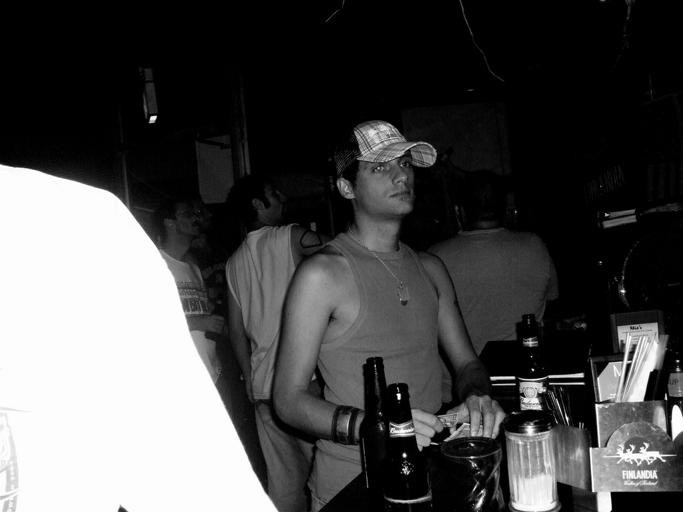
[137,67,160,126]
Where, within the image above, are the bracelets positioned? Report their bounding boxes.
[328,401,361,447]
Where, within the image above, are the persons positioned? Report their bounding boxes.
[272,118,509,511]
[139,152,584,510]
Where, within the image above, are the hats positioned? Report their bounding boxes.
[334,120,438,179]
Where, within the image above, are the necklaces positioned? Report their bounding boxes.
[351,229,411,306]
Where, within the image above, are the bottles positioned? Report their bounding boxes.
[500,409,560,511]
[664,357,683,406]
[358,355,434,511]
[513,311,551,412]
[205,298,227,340]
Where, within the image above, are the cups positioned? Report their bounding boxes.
[441,437,505,512]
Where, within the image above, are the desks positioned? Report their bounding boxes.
[304,341,682,512]
